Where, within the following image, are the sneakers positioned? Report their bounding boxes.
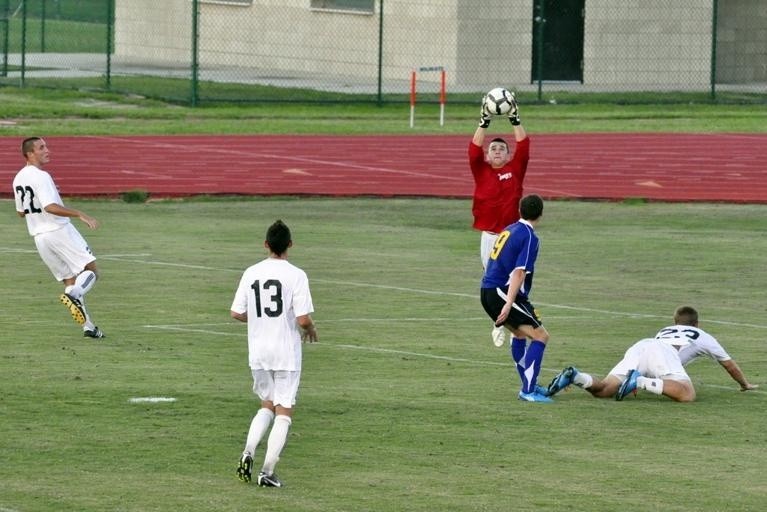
[614,369,640,401]
[519,367,578,404]
[257,471,283,486]
[491,325,506,348]
[60,293,86,325]
[83,328,105,339]
[237,451,253,483]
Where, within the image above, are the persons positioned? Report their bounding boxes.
[547,306,760,402]
[479,195,554,402]
[12,137,106,338]
[468,92,530,348]
[230,219,319,487]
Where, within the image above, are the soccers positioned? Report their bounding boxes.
[486,88,514,117]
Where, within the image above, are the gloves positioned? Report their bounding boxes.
[480,97,492,127]
[507,100,521,126]
